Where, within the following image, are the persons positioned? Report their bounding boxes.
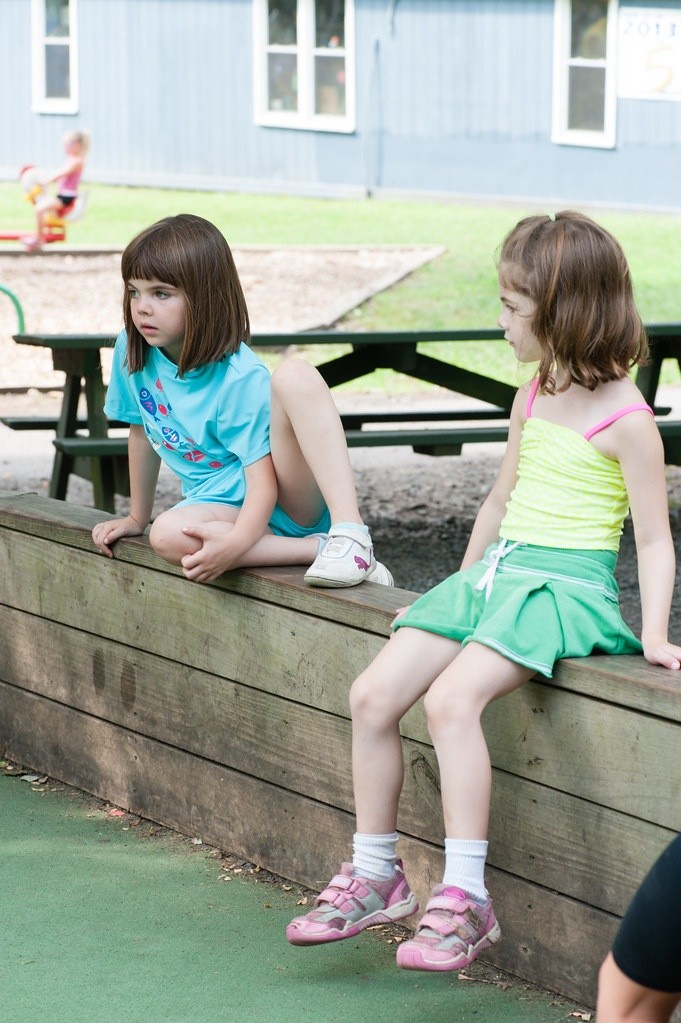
[595,833,681,1023]
[286,209,681,971]
[21,131,89,246]
[92,215,394,587]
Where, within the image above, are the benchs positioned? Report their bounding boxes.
[51,420,681,455]
[0,406,672,432]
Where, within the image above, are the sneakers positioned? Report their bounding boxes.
[396,883,501,971]
[286,858,419,946]
[303,528,376,588]
[306,534,396,588]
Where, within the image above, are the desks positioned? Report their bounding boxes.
[12,321,681,515]
